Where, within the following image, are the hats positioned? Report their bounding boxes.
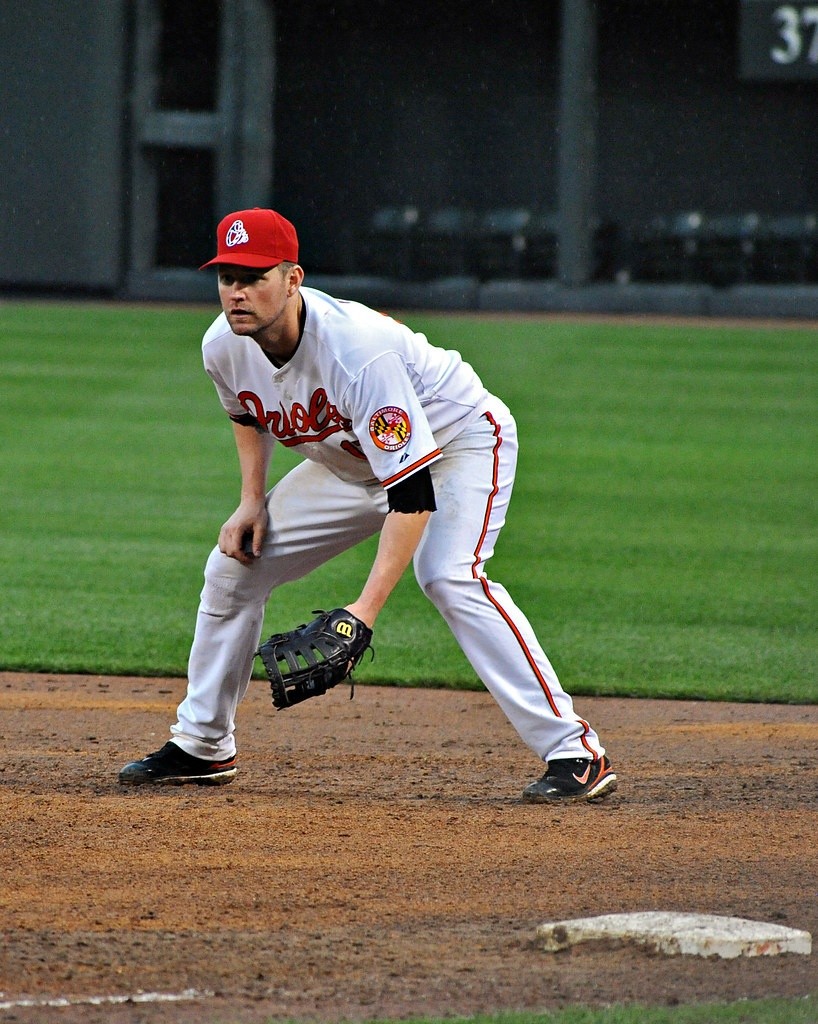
[197,208,299,272]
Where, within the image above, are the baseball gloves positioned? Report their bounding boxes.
[251,612,378,710]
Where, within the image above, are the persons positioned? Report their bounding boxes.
[120,207,617,803]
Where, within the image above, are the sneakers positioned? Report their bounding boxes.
[117,739,237,789]
[523,754,615,802]
